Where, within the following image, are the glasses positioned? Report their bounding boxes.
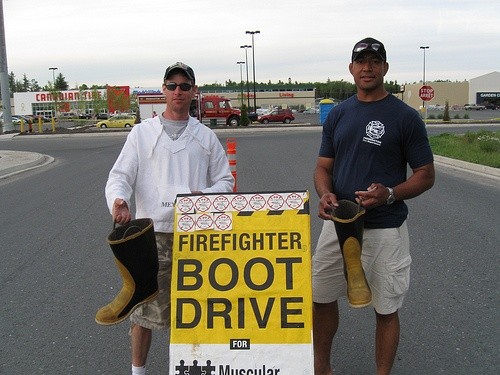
[164,82,194,91]
[354,43,381,52]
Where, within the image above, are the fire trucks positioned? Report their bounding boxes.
[137,92,241,128]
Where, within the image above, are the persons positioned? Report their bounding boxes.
[310,37,435,375]
[104,61,235,375]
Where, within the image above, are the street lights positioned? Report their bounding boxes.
[246,30,261,114]
[419,46,429,108]
[237,61,245,110]
[240,45,252,109]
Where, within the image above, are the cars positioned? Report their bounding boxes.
[11,110,138,124]
[97,115,136,128]
[249,108,295,124]
[484,103,499,110]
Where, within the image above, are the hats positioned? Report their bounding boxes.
[352,37,386,64]
[164,62,195,86]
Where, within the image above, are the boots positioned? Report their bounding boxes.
[95,213,160,325]
[329,196,373,309]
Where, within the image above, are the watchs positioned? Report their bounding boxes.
[386,187,396,205]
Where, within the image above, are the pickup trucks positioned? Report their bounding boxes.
[463,103,486,111]
